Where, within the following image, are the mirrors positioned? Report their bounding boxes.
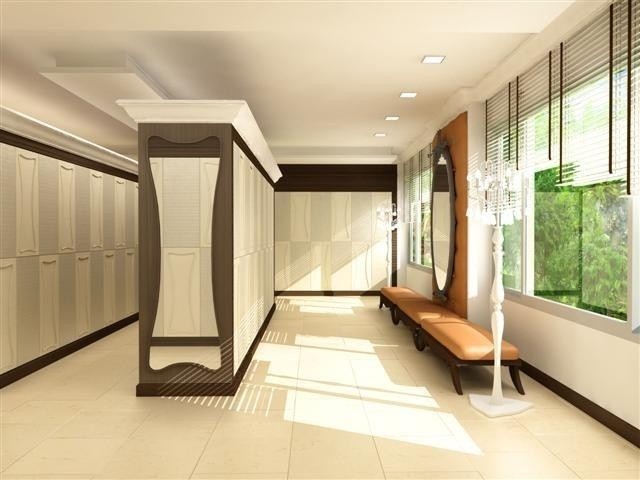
[428,136,457,302]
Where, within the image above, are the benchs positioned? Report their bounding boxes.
[373,286,526,397]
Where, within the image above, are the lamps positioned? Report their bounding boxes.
[464,158,537,420]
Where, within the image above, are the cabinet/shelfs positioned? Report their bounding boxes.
[273,157,400,300]
[0,105,139,387]
[115,98,282,397]
